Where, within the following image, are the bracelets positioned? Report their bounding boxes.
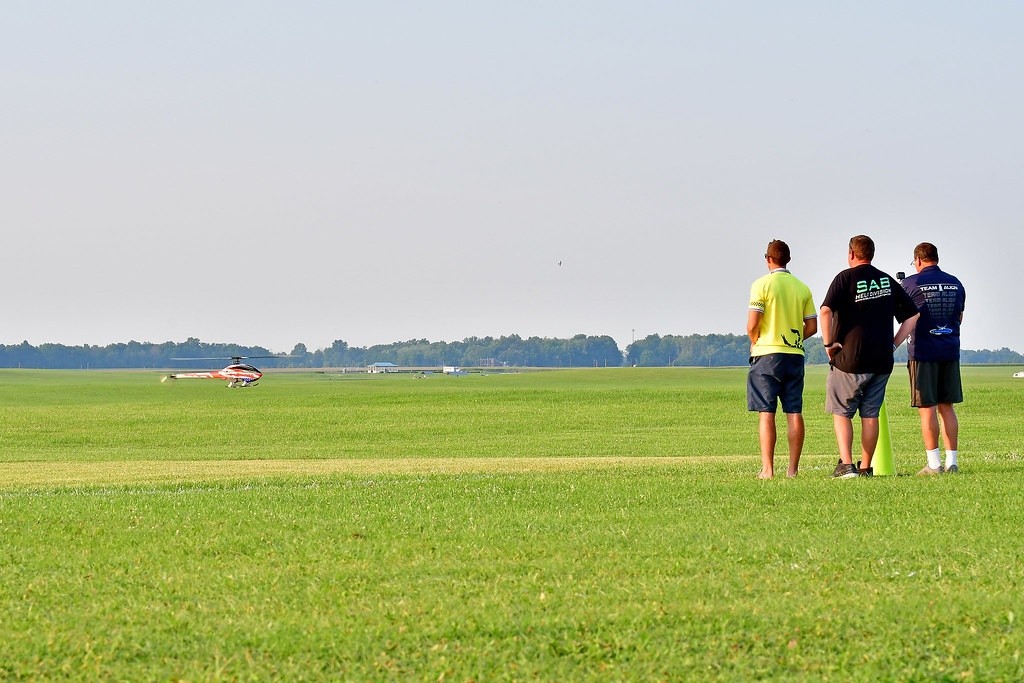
[893,343,896,352]
[823,341,833,347]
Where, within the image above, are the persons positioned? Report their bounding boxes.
[819,234,920,477]
[746,238,818,481]
[901,243,966,476]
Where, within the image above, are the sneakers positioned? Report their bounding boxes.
[857,461,874,478]
[830,460,857,480]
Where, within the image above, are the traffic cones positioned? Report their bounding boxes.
[872,399,899,476]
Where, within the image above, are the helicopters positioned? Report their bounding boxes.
[159,355,305,389]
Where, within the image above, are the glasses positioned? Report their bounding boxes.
[765,254,772,259]
[910,260,916,266]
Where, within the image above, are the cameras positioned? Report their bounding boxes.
[896,272,905,279]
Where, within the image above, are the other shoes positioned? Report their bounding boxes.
[917,465,944,475]
[944,465,957,474]
